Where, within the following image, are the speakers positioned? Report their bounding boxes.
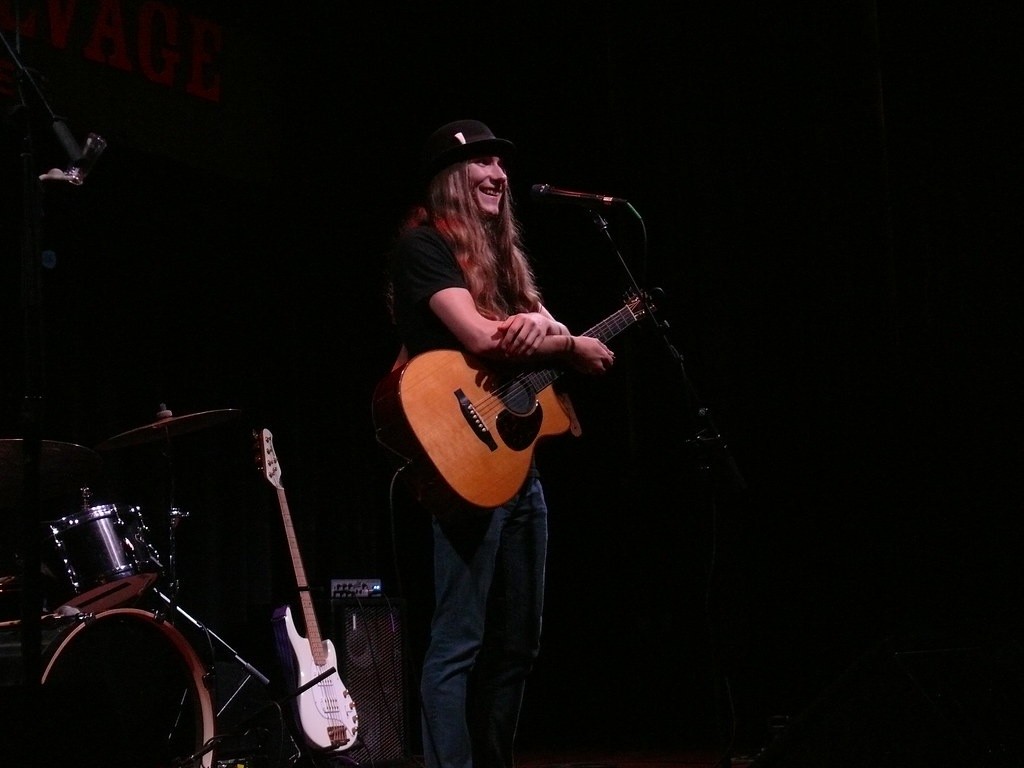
[315,595,409,768]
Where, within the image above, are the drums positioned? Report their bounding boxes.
[35,608,217,768]
[33,503,163,614]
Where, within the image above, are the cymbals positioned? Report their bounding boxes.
[96,409,241,451]
[0,438,104,489]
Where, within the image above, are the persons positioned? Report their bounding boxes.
[394,115,615,768]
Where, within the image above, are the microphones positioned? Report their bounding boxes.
[530,183,629,208]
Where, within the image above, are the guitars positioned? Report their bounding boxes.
[371,283,662,525]
[253,426,360,754]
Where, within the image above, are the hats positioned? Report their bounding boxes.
[425,120,517,178]
